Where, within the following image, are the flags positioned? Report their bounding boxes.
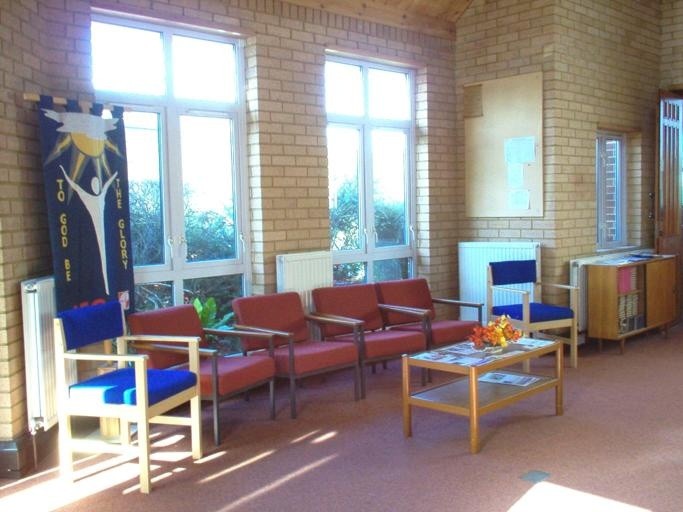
[35,93,136,321]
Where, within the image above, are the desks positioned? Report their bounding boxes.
[401,337,564,454]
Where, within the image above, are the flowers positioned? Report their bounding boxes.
[464,314,524,352]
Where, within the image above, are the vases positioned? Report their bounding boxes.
[482,346,502,355]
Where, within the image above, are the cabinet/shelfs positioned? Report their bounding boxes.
[582,255,677,354]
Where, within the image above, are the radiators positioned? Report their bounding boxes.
[457,241,541,327]
[273,250,335,344]
[19,274,63,437]
[569,248,655,346]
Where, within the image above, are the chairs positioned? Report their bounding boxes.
[229,278,484,419]
[485,258,579,374]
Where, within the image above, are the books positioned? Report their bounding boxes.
[593,249,673,333]
